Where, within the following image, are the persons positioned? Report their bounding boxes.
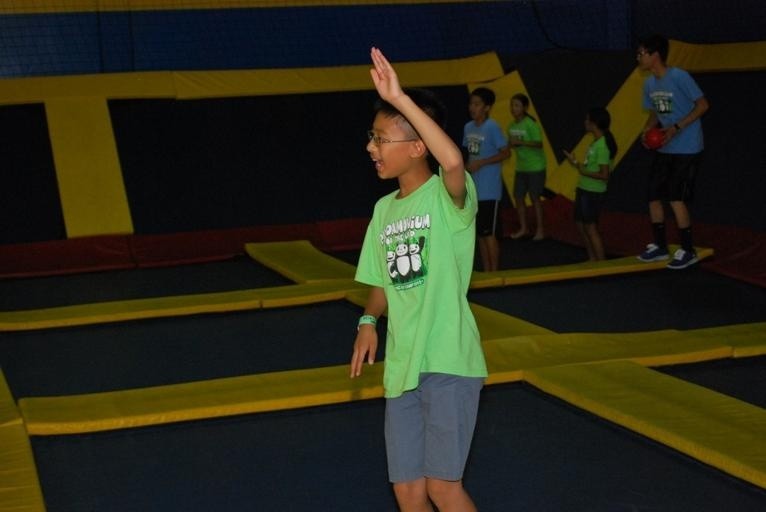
[563,107,617,262]
[636,34,710,270]
[350,47,489,512]
[461,87,547,272]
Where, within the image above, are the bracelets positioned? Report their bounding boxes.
[357,314,377,331]
[674,124,680,130]
[572,160,577,165]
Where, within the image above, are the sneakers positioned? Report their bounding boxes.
[666,249,698,270]
[637,244,669,263]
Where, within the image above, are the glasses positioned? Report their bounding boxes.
[367,130,418,144]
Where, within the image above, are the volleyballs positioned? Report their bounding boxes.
[644,128,665,149]
